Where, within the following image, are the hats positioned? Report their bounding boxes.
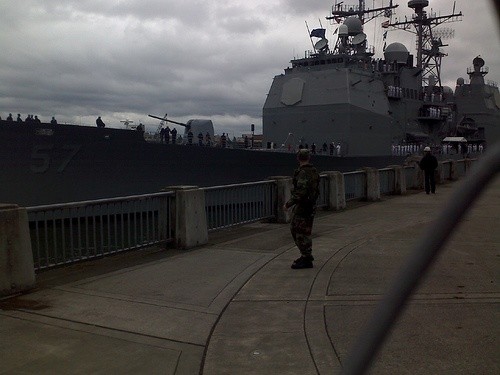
[458,141,462,144]
[297,148,310,156]
[422,146,432,151]
[337,143,340,145]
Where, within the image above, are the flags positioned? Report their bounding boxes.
[310,29,325,39]
[384,10,390,18]
[332,28,337,35]
[382,20,389,28]
[383,31,387,40]
[383,42,386,51]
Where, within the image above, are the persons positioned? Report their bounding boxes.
[136,123,228,147]
[370,57,397,71]
[96,116,106,127]
[299,142,341,155]
[419,147,438,194]
[284,149,320,269]
[244,136,249,148]
[393,143,483,154]
[419,106,440,118]
[7,113,57,124]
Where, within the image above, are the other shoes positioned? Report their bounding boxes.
[426,191,430,194]
[431,190,435,193]
[291,255,314,269]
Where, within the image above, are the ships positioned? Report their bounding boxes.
[0,0,500,265]
